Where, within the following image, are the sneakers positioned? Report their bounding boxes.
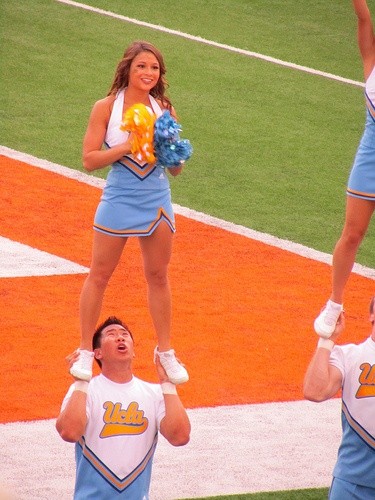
[314,298,344,338]
[153,345,188,385]
[69,348,96,382]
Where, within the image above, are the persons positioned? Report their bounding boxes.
[69,39,189,385]
[303,303,375,500]
[313,0,375,338]
[55,316,191,500]
